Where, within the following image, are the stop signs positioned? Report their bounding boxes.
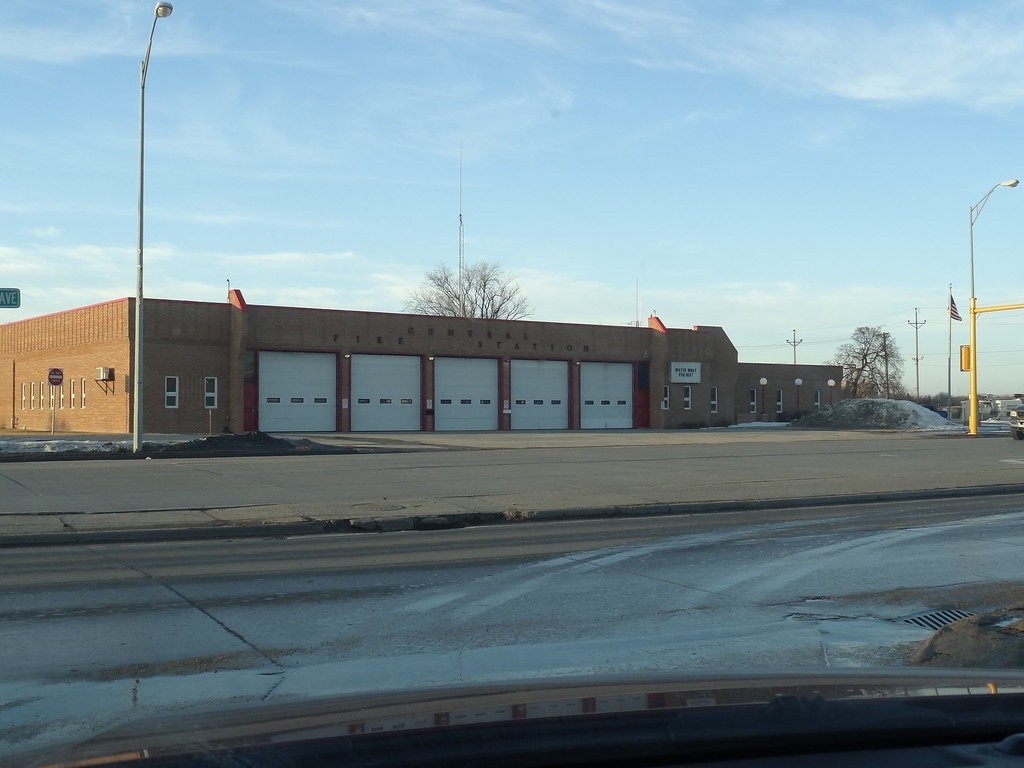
[48,368,64,386]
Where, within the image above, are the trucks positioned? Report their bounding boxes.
[978,399,1022,416]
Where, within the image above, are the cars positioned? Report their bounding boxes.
[1009,404,1024,440]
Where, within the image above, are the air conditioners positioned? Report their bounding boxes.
[94,366,109,380]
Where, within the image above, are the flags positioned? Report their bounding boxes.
[948,295,962,321]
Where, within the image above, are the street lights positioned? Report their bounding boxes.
[759,378,769,413]
[133,1,195,453]
[969,179,1019,297]
[795,378,803,413]
[827,379,835,404]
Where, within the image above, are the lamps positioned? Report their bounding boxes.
[344,353,351,359]
[426,356,435,361]
[575,361,582,366]
